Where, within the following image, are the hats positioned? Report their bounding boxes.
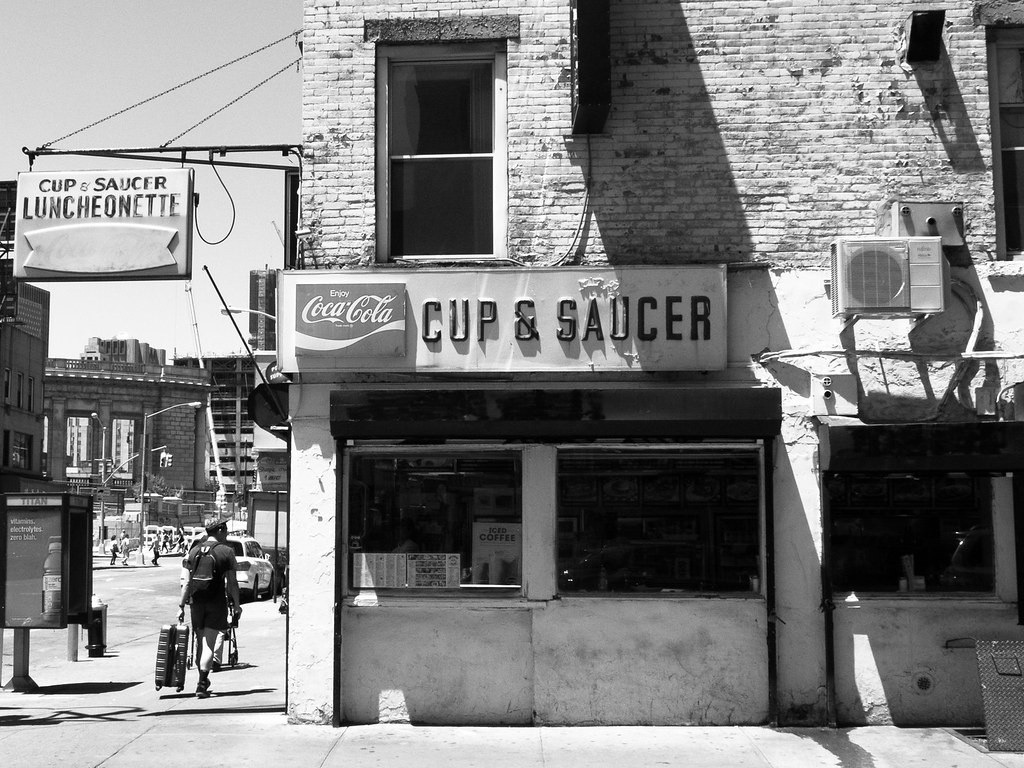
[205,518,230,531]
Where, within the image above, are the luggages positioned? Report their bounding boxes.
[154,618,189,692]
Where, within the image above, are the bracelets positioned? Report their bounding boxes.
[180,604,185,609]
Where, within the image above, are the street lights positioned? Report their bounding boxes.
[91,411,108,544]
[139,401,202,553]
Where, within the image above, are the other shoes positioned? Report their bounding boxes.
[195,678,210,697]
[212,661,221,672]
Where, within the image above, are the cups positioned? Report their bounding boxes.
[487,554,518,590]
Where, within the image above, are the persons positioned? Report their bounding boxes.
[390,519,420,553]
[179,516,243,699]
[109,527,189,567]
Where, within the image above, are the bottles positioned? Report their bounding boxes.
[43,542,60,622]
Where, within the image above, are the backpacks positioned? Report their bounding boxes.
[190,540,222,601]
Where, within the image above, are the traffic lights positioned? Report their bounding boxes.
[160,452,166,468]
[166,453,173,468]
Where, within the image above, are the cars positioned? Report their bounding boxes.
[179,534,275,602]
